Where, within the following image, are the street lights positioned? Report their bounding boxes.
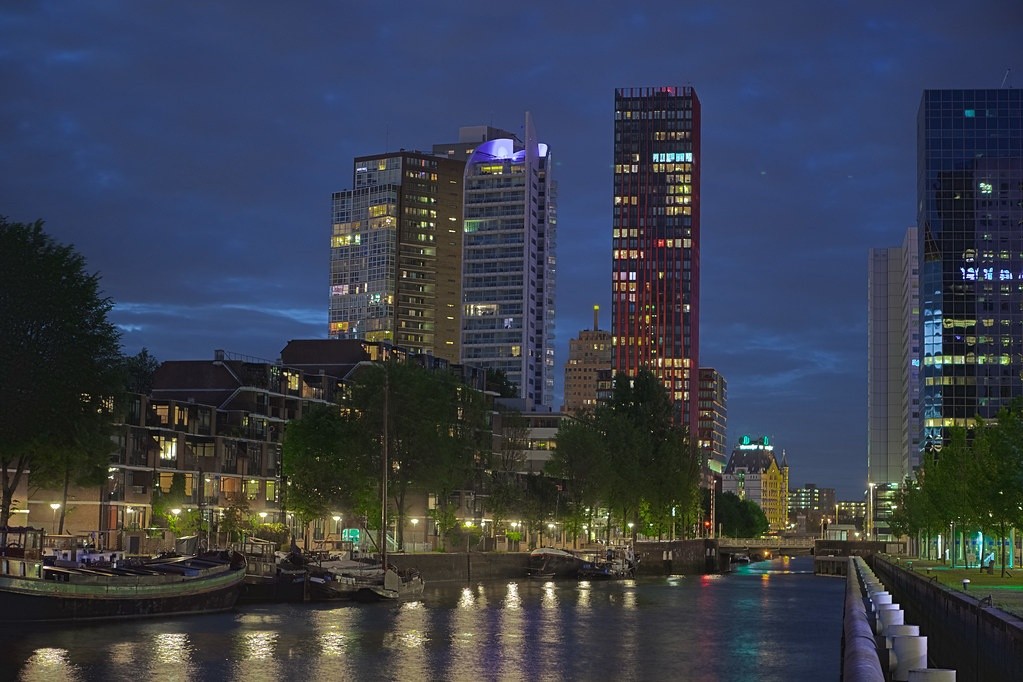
[466,521,472,553]
[259,512,267,536]
[411,518,419,553]
[171,508,181,552]
[332,515,341,541]
[628,523,634,538]
[511,522,517,552]
[50,504,60,535]
[547,524,555,547]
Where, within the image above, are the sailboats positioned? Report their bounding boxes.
[300,359,425,606]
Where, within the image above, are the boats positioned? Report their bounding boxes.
[527,572,556,579]
[530,539,648,581]
[238,536,313,588]
[0,549,247,624]
